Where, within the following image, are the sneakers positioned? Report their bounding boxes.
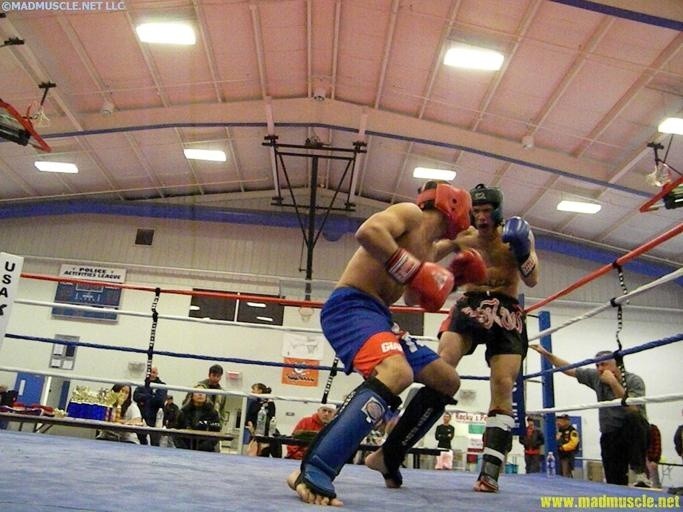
[633,472,654,488]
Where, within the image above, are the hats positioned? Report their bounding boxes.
[557,414,568,418]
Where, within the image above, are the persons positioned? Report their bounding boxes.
[673,425,683,461]
[93,365,402,466]
[555,413,580,479]
[526,343,654,488]
[519,415,545,474]
[644,424,662,488]
[286,177,490,506]
[434,411,455,470]
[365,182,540,493]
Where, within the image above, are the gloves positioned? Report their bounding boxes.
[385,248,455,312]
[447,248,488,292]
[502,216,537,277]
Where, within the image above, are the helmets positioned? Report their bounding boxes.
[416,180,473,240]
[470,184,505,229]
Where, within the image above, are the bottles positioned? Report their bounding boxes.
[545,451,557,479]
[255,406,266,437]
[155,408,166,428]
[268,414,277,438]
[123,406,132,425]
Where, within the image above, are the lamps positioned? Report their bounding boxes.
[521,135,534,149]
[314,86,325,102]
[100,99,114,118]
